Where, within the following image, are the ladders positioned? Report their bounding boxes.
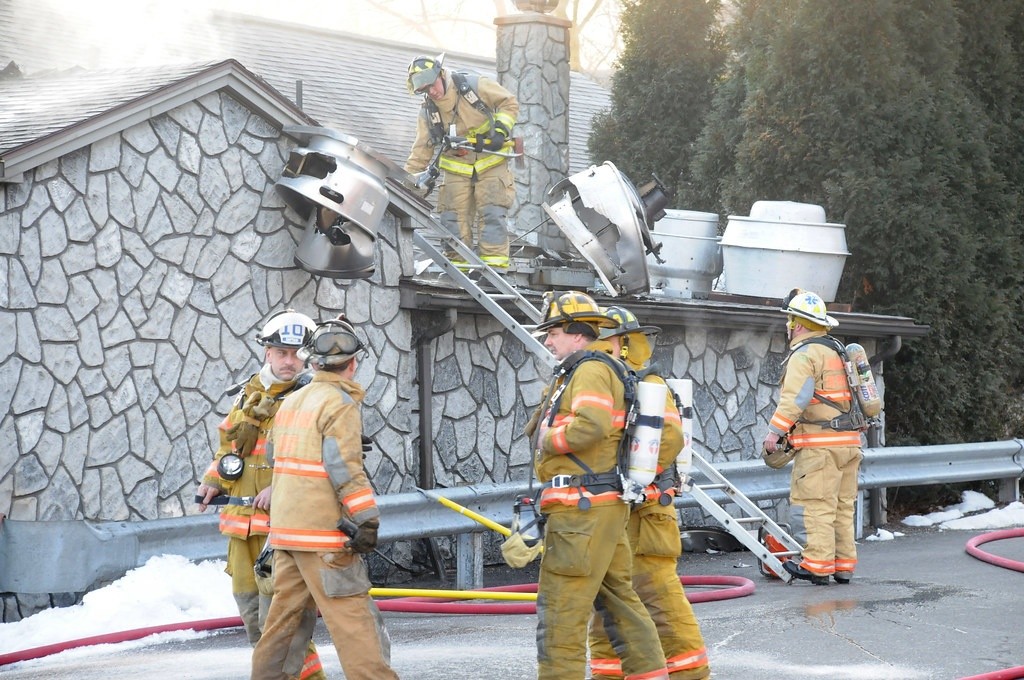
[413,215,814,586]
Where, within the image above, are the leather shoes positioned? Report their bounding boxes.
[478,273,508,287]
[782,560,829,585]
[438,271,469,282]
[831,574,850,585]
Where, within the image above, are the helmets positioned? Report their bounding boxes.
[407,52,444,94]
[599,306,662,339]
[297,313,367,364]
[780,288,839,326]
[536,291,620,331]
[256,308,317,349]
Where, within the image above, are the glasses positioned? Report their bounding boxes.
[313,332,359,355]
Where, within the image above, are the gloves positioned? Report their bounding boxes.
[485,132,504,151]
[226,407,267,457]
[343,519,380,553]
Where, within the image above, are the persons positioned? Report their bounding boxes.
[761,291,867,585]
[250,314,402,680]
[522,294,710,680]
[403,55,520,288]
[195,310,326,680]
